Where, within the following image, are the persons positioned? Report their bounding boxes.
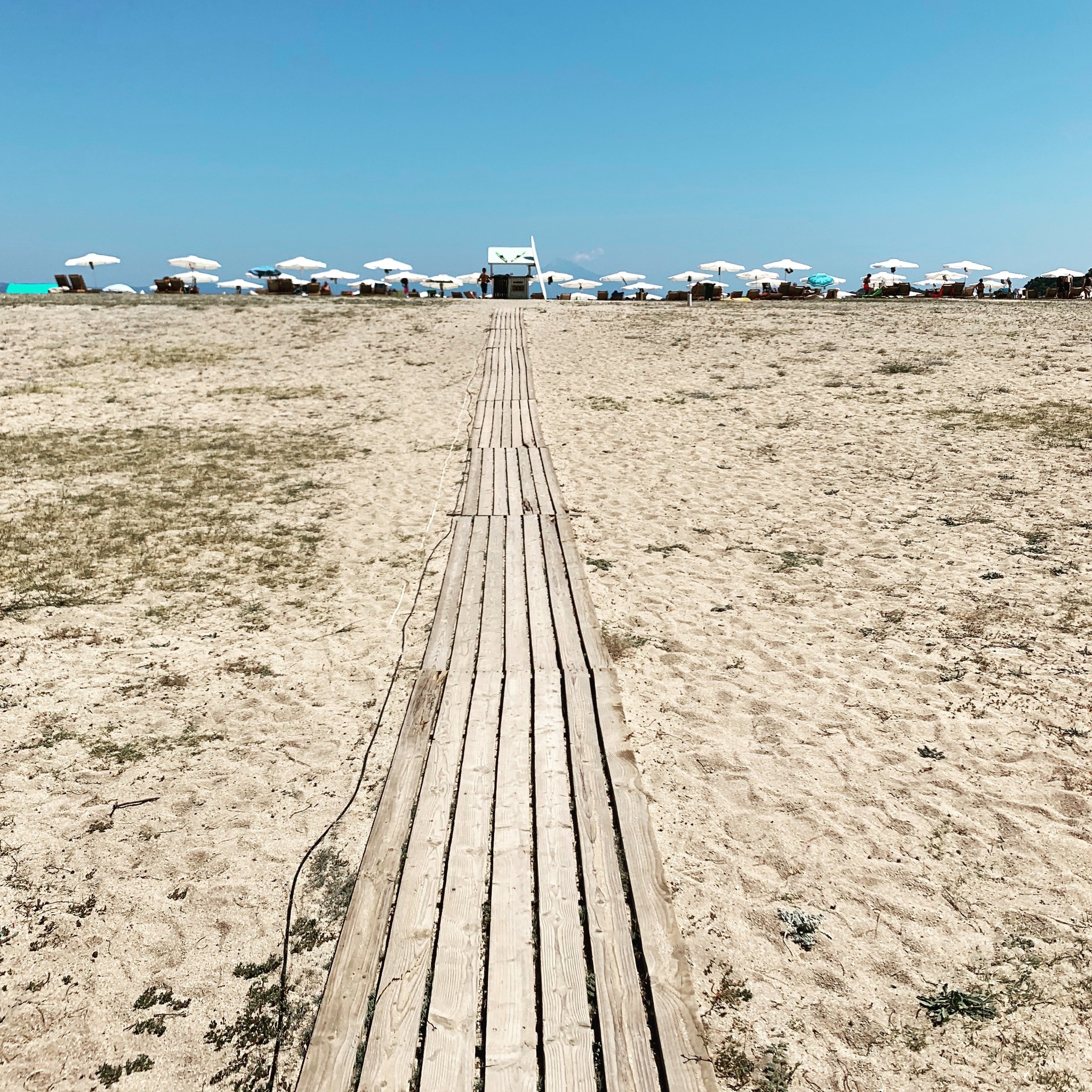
[400,278,410,298]
[807,286,811,292]
[477,268,490,299]
[1013,288,1019,298]
[863,274,872,295]
[975,279,985,299]
[1082,273,1092,300]
[1056,275,1073,299]
[793,282,797,286]
[306,278,329,294]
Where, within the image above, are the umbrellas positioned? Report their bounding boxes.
[558,279,603,301]
[621,283,663,303]
[381,271,493,299]
[529,271,574,299]
[668,260,857,299]
[64,253,137,294]
[363,258,413,271]
[860,258,1028,296]
[346,279,393,289]
[1040,268,1085,299]
[167,255,264,294]
[599,272,647,287]
[246,256,360,285]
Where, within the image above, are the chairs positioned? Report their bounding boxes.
[532,291,650,301]
[342,283,478,299]
[154,277,200,295]
[850,282,1084,300]
[255,278,331,296]
[55,274,102,293]
[666,282,838,301]
